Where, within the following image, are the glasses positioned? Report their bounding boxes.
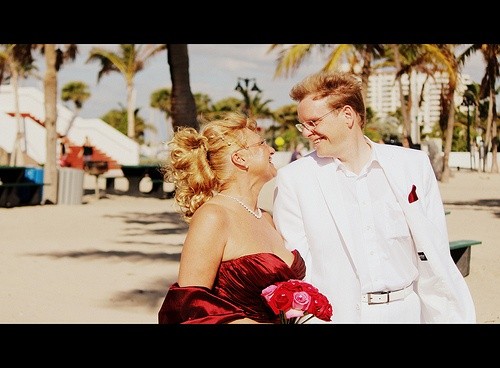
[295,106,343,133]
[231,137,267,156]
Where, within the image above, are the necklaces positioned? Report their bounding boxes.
[215,192,263,219]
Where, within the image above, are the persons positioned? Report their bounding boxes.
[272,73,477,324]
[469,136,477,170]
[59,135,66,160]
[478,139,488,173]
[78,135,94,168]
[156,111,316,324]
[288,142,304,163]
[377,127,421,149]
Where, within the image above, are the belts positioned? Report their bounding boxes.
[361,282,414,305]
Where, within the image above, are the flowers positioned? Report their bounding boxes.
[260,278,333,324]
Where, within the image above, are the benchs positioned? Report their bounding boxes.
[0,182,52,206]
[99,175,124,191]
[449,239,482,278]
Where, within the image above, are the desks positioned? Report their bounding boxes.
[0,166,34,184]
[120,165,164,197]
